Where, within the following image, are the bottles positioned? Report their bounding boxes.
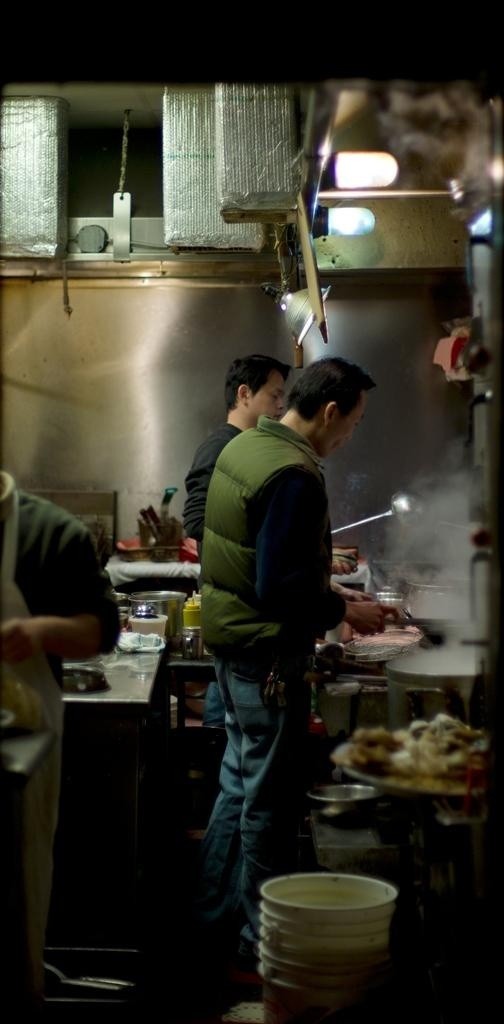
[181,626,200,659]
[182,590,200,628]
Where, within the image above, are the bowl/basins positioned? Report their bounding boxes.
[309,784,381,827]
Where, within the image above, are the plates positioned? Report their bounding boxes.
[343,767,468,799]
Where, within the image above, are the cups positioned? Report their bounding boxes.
[257,872,398,1021]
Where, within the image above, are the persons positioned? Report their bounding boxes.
[183,354,291,769]
[199,357,373,974]
[0,469,121,995]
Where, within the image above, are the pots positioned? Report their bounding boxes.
[386,652,474,728]
[406,577,467,620]
[110,590,186,637]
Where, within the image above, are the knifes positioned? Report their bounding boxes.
[139,485,177,523]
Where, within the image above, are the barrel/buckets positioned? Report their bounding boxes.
[256,873,399,1024]
